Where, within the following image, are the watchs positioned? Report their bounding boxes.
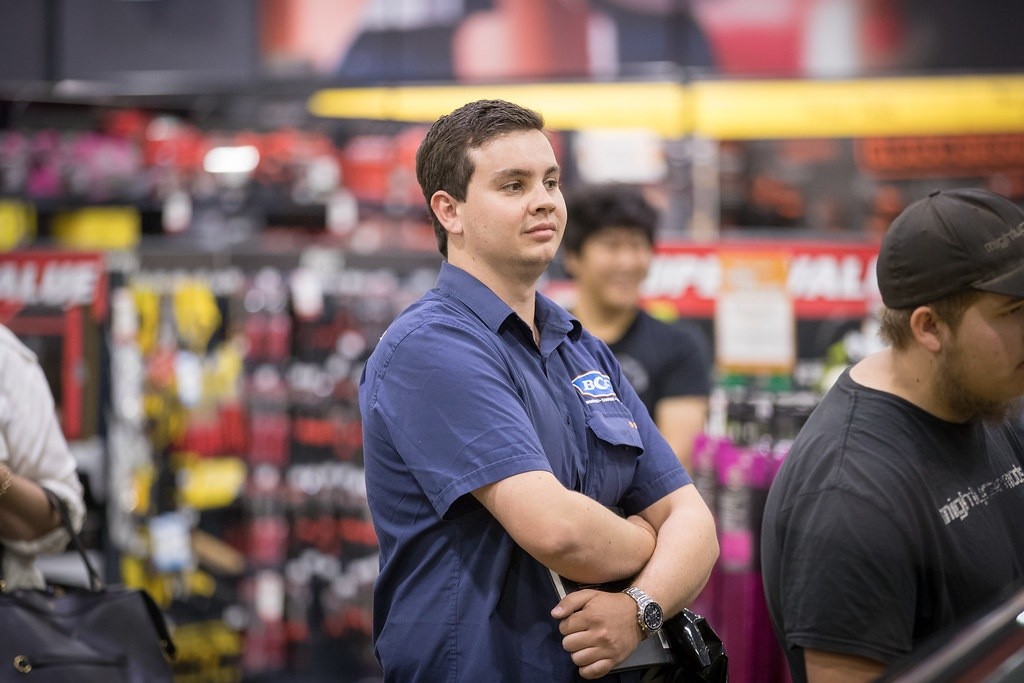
[622,587,664,641]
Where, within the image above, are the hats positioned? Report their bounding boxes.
[876,188,1024,308]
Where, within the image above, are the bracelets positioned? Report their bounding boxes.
[0,464,14,495]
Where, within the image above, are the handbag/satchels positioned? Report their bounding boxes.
[0,487,177,683]
[640,608,728,683]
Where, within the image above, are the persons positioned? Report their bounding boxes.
[0,324,87,591]
[761,187,1024,683]
[359,99,719,683]
[557,188,712,474]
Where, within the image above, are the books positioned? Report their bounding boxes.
[547,569,673,669]
